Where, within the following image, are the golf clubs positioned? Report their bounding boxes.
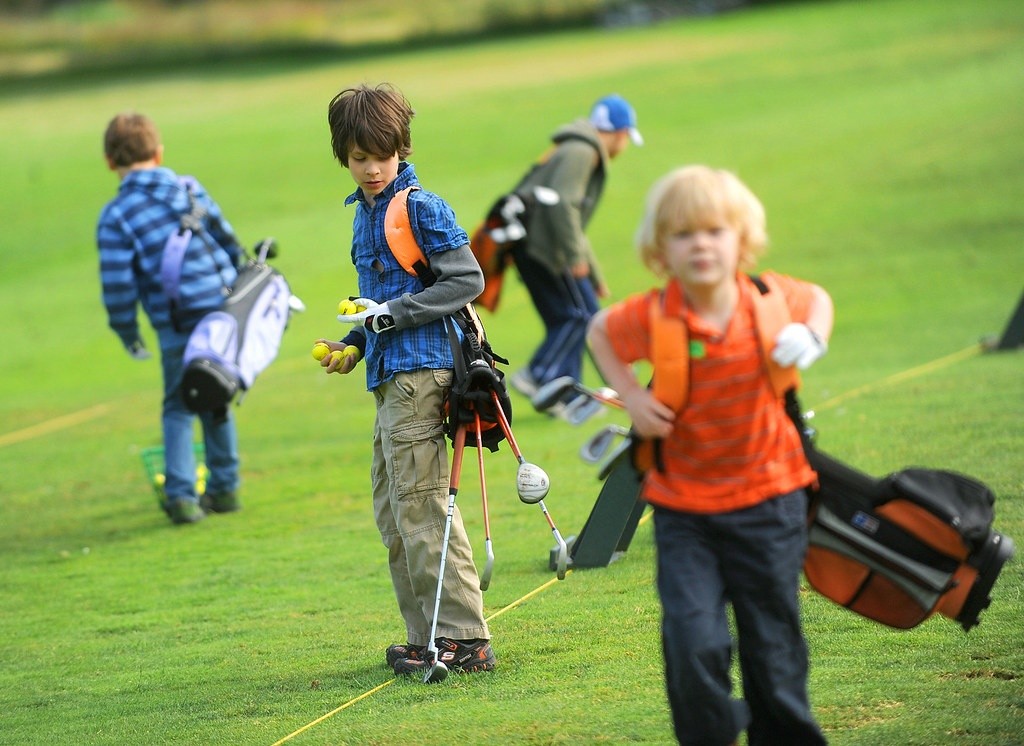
[488,387,568,581]
[474,410,495,591]
[491,186,561,241]
[529,375,633,463]
[422,423,466,684]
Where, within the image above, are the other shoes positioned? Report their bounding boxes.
[197,492,239,513]
[160,492,206,523]
[511,368,606,423]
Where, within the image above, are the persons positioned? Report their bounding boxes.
[586,164,835,746]
[96,115,249,524]
[315,88,494,678]
[509,96,644,415]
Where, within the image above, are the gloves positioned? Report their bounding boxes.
[770,322,827,370]
[127,338,153,359]
[337,296,396,334]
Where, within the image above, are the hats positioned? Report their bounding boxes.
[590,94,644,147]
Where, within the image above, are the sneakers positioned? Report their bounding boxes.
[394,637,495,677]
[387,641,428,666]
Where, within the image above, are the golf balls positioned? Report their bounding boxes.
[312,342,331,361]
[154,460,209,497]
[343,345,361,363]
[338,300,357,315]
[328,350,346,369]
[356,305,366,313]
